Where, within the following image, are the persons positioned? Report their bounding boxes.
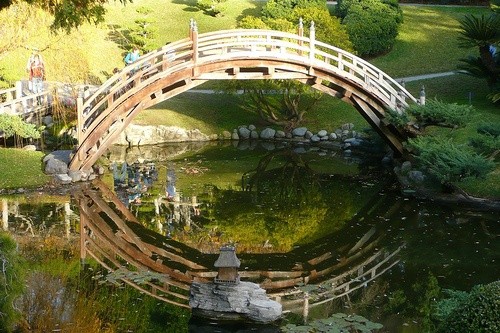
[124,45,141,89]
[28,54,46,105]
